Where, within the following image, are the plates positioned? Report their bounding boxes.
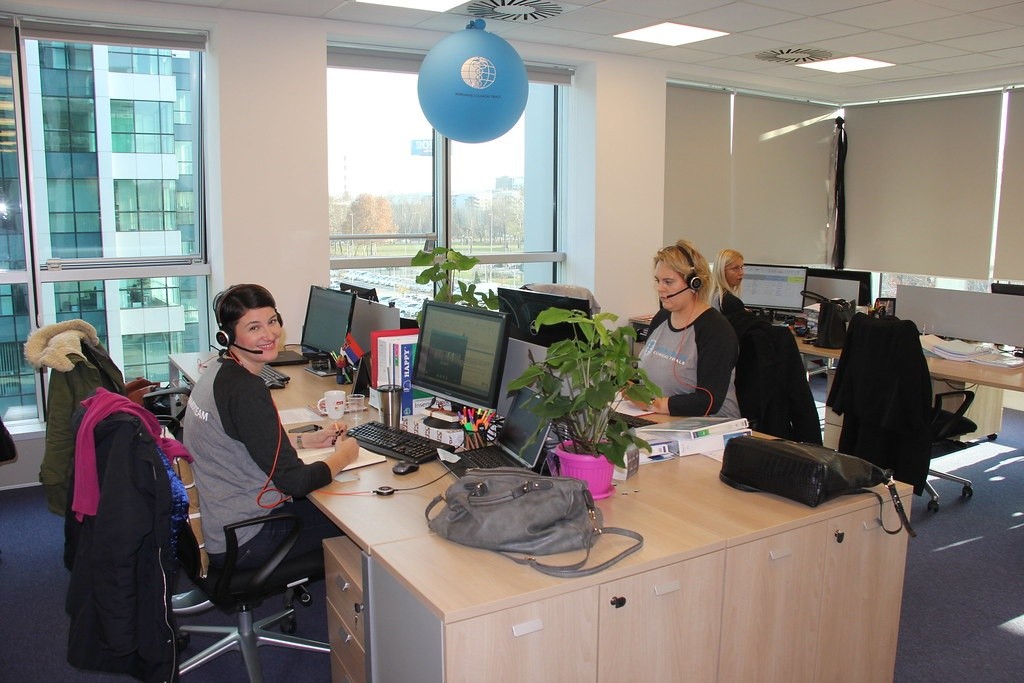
[592,487,616,500]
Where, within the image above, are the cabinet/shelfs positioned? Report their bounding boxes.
[824,368,844,452]
[323,536,367,683]
[367,472,913,683]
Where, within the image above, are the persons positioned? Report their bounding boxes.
[708,248,746,316]
[622,239,742,421]
[183,283,360,569]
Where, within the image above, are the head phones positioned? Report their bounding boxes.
[212,284,283,347]
[676,244,705,290]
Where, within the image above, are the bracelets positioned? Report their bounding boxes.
[297,433,304,449]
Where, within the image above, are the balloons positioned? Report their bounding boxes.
[417,29,528,144]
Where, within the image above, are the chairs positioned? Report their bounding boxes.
[842,313,978,512]
[50,329,331,683]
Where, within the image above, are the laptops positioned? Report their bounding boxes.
[436,387,553,482]
[266,350,309,366]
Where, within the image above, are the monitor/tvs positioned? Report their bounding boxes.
[300,283,378,377]
[409,287,600,414]
[735,264,808,322]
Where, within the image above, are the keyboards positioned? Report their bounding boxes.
[345,421,456,464]
[258,365,290,383]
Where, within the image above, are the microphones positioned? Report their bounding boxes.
[232,344,263,354]
[666,286,691,298]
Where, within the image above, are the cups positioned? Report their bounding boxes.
[347,394,365,422]
[317,390,346,420]
[377,384,404,429]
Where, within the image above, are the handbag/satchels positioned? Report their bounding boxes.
[719,435,919,538]
[799,290,855,348]
[424,467,645,578]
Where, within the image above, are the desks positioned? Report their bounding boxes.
[171,346,782,555]
[626,315,1024,444]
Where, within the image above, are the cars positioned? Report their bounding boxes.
[330,269,467,297]
[478,263,523,277]
[330,278,424,317]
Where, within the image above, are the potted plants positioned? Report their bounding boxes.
[505,307,665,501]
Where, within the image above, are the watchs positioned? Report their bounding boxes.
[647,396,655,411]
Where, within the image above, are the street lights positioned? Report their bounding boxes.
[349,213,354,250]
[488,213,493,253]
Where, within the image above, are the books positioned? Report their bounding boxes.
[932,340,1024,369]
[627,314,657,325]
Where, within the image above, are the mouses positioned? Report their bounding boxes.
[265,381,285,389]
[392,460,419,475]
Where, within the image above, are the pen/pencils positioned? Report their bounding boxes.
[334,421,340,432]
[330,350,352,383]
[457,406,496,450]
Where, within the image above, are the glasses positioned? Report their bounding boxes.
[727,265,747,272]
